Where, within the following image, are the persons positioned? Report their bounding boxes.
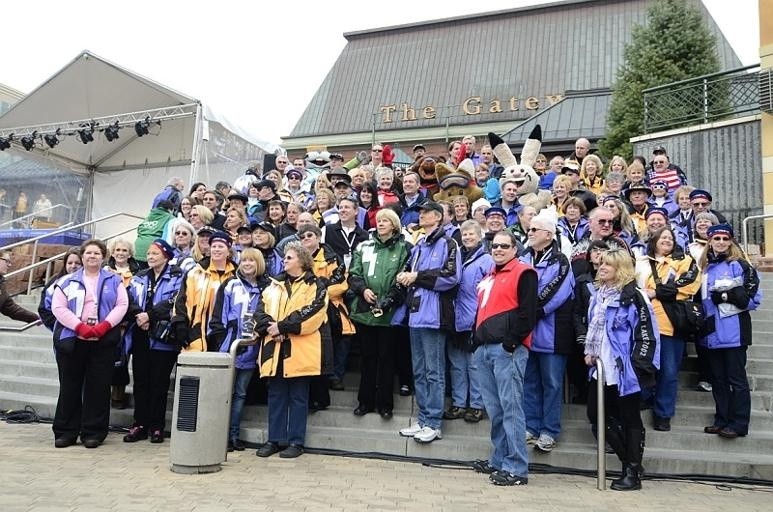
[0,182,10,218]
[31,192,53,223]
[464,231,541,485]
[10,190,29,229]
[0,249,44,327]
[36,132,761,460]
[579,248,655,492]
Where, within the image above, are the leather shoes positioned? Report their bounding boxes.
[704,424,739,438]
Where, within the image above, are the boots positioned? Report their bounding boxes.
[605,424,645,491]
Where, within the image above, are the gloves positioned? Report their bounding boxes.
[75,320,112,339]
[709,291,728,305]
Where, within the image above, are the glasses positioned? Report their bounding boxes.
[527,228,543,232]
[492,244,510,249]
[372,150,382,152]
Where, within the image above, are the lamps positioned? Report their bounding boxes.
[0,118,158,152]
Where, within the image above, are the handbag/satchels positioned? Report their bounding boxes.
[672,300,715,341]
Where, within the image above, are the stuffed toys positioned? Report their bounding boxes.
[487,124,544,206]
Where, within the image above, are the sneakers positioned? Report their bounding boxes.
[124,422,163,443]
[640,395,671,431]
[256,442,278,457]
[399,424,442,443]
[227,440,245,452]
[279,444,304,458]
[331,379,345,390]
[698,382,712,391]
[354,406,374,415]
[380,408,392,418]
[113,400,124,410]
[55,436,99,449]
[526,430,556,452]
[473,459,528,485]
[444,406,482,422]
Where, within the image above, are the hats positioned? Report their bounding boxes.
[483,207,507,220]
[561,145,733,238]
[154,152,352,260]
[412,144,426,152]
[413,201,443,214]
